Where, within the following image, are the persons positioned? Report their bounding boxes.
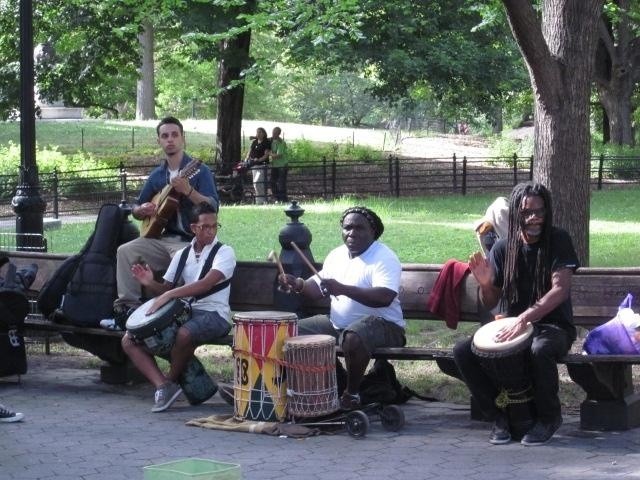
[245,127,271,205]
[99,117,220,331]
[277,206,407,413]
[452,181,580,445]
[120,201,237,413]
[249,127,288,203]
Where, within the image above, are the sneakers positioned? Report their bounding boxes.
[488,421,511,444]
[521,411,563,446]
[100,319,122,330]
[151,381,180,413]
[340,391,360,411]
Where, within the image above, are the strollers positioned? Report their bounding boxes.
[210,160,257,208]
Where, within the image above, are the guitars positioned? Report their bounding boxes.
[140,157,203,238]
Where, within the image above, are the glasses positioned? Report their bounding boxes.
[197,224,222,231]
[520,209,544,217]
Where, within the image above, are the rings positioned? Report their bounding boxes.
[324,287,328,294]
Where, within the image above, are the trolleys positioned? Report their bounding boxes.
[288,401,407,438]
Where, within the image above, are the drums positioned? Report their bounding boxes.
[125,295,218,404]
[282,334,340,417]
[471,317,540,441]
[231,311,298,422]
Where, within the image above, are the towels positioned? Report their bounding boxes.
[426,255,471,330]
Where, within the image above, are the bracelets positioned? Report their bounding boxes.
[295,277,305,294]
[531,302,544,323]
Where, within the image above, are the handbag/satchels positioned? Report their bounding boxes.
[583,294,640,354]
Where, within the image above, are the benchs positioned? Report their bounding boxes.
[336,264,640,431]
[0,250,325,385]
